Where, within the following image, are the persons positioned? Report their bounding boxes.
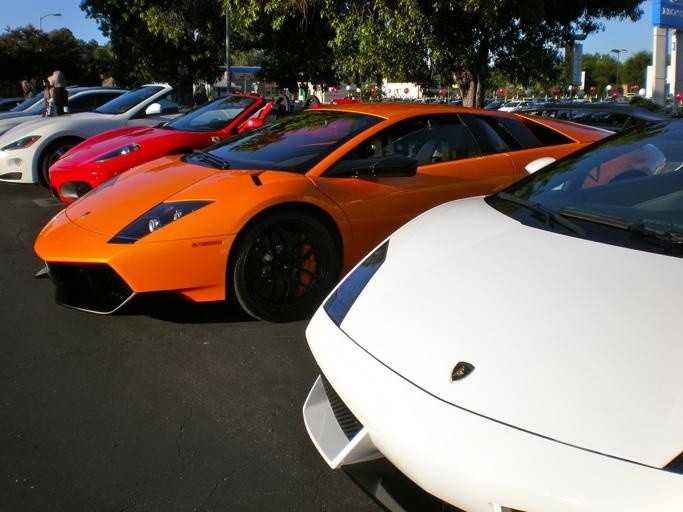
[45,70,69,116]
[181,94,200,112]
[21,79,36,101]
[99,68,118,88]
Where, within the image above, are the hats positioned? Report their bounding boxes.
[48,71,67,87]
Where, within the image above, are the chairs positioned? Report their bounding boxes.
[262,96,320,120]
[364,115,471,165]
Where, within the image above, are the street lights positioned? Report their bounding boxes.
[39,13,61,30]
[610,49,628,85]
